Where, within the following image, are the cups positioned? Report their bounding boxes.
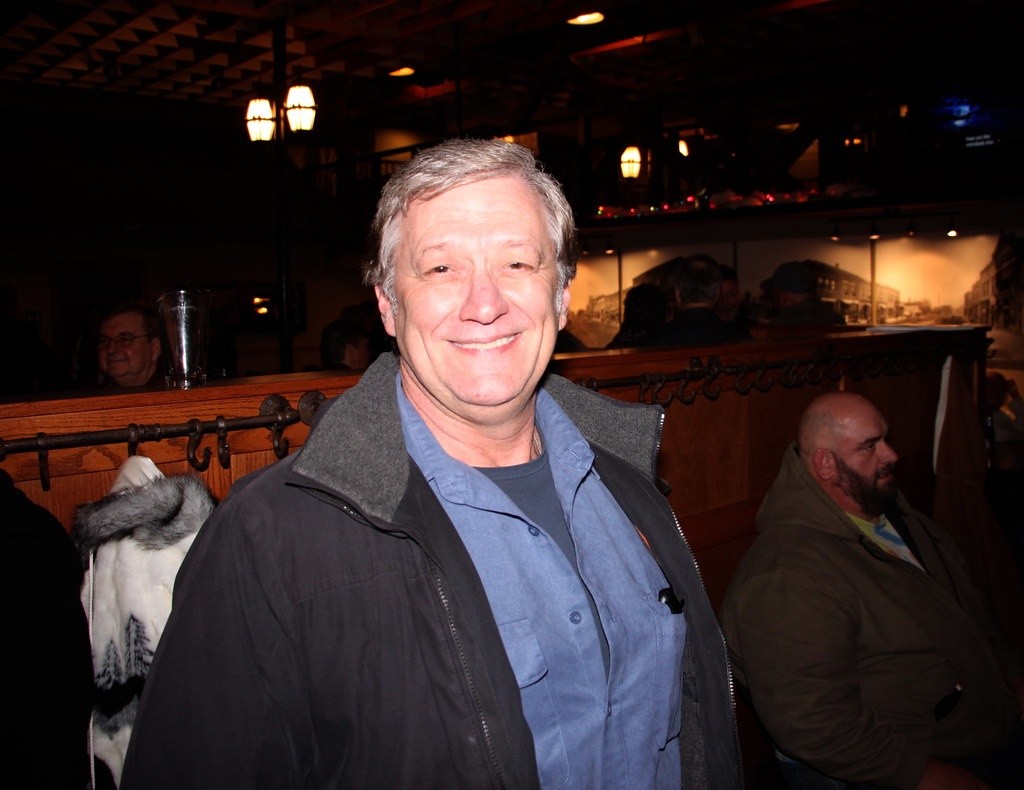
[158,288,208,386]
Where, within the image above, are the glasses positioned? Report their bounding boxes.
[93,334,149,349]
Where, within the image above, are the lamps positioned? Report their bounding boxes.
[620,143,641,179]
[287,82,315,131]
[246,97,273,146]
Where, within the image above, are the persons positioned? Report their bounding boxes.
[0,462,96,790]
[118,140,748,790]
[724,393,1024,790]
[92,303,173,396]
[985,372,1022,440]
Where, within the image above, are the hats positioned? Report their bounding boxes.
[760,261,815,292]
[682,252,737,283]
